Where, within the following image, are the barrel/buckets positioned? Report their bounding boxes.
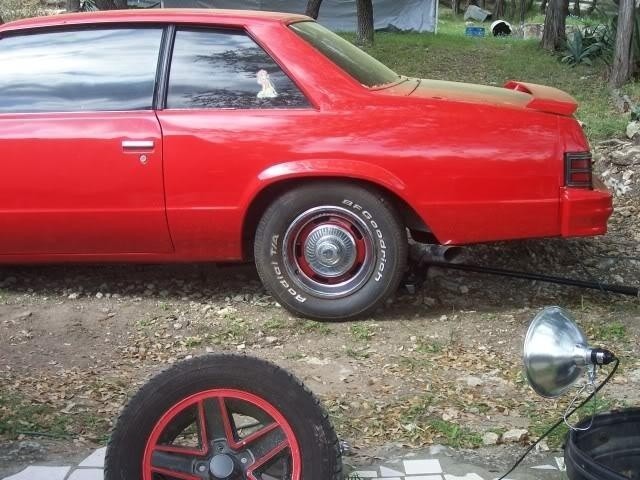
[562,407,640,480]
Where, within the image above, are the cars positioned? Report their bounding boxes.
[0,8,612,321]
